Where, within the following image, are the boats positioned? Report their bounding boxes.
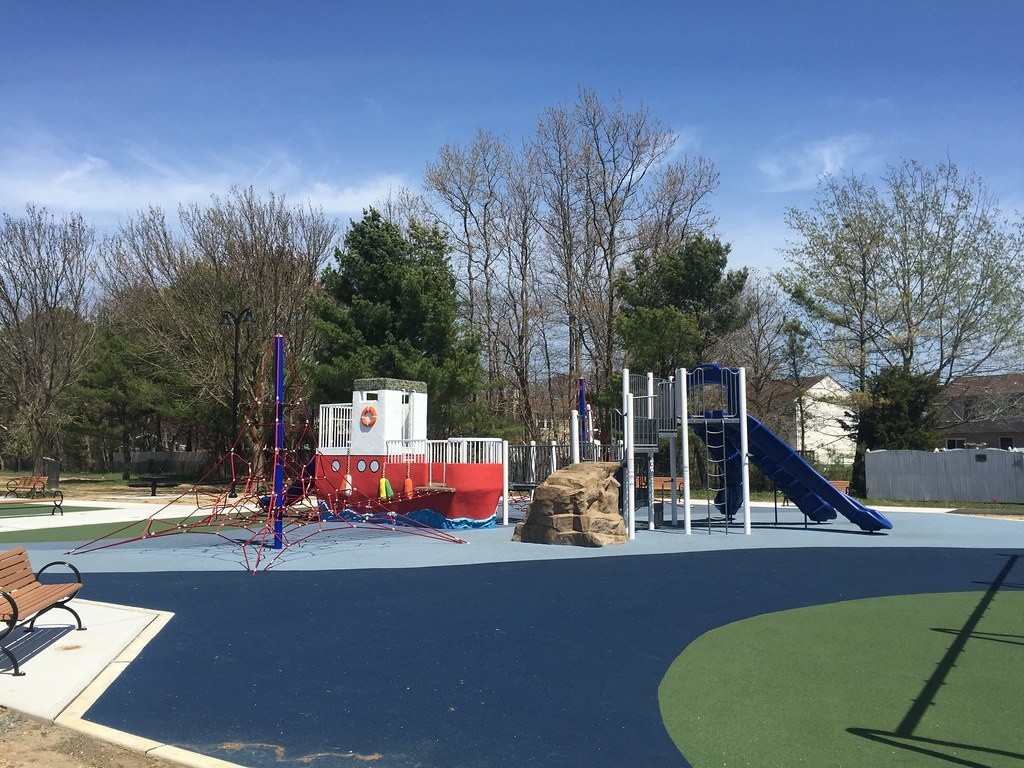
[314,377,504,531]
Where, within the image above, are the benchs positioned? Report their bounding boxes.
[5,476,48,499]
[0,546,88,677]
[0,491,64,517]
[782,480,850,507]
[635,476,684,502]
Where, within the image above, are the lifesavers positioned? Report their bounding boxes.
[360,406,377,428]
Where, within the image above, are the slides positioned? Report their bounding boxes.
[690,410,894,532]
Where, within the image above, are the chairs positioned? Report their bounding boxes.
[260,479,315,507]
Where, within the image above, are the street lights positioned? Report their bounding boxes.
[219,307,256,498]
